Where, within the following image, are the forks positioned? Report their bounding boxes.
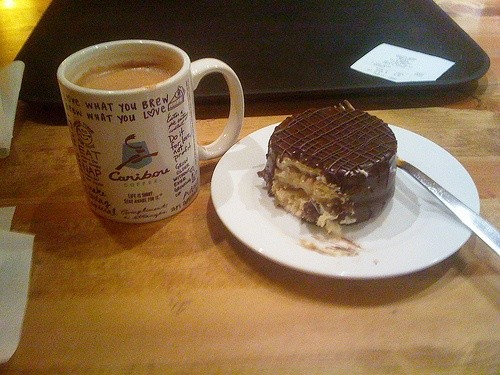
[333,99,500,257]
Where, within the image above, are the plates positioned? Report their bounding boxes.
[210,122,480,279]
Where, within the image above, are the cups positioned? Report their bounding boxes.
[57,39,245,223]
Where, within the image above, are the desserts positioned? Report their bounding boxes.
[257,107,398,231]
